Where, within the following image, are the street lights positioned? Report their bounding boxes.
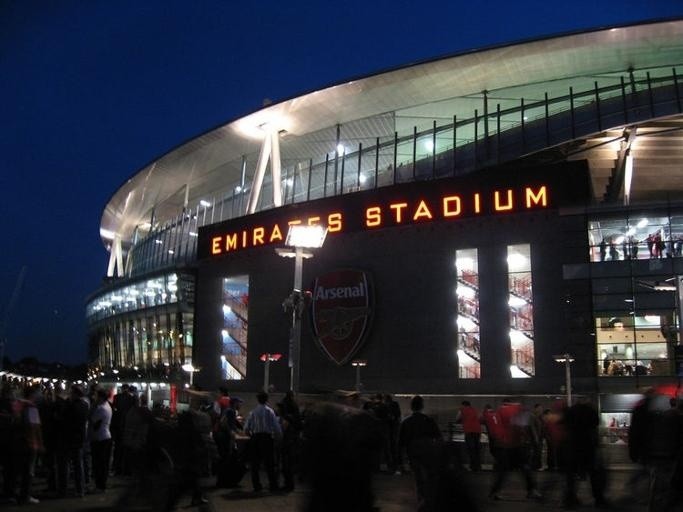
[348,358,367,390]
[281,223,330,393]
[554,357,575,402]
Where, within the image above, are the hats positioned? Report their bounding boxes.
[410,396,424,412]
[219,396,245,408]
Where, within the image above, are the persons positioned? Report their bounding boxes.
[590,224,683,260]
[0,360,682,511]
[601,348,652,379]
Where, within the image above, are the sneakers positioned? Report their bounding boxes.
[19,496,39,504]
